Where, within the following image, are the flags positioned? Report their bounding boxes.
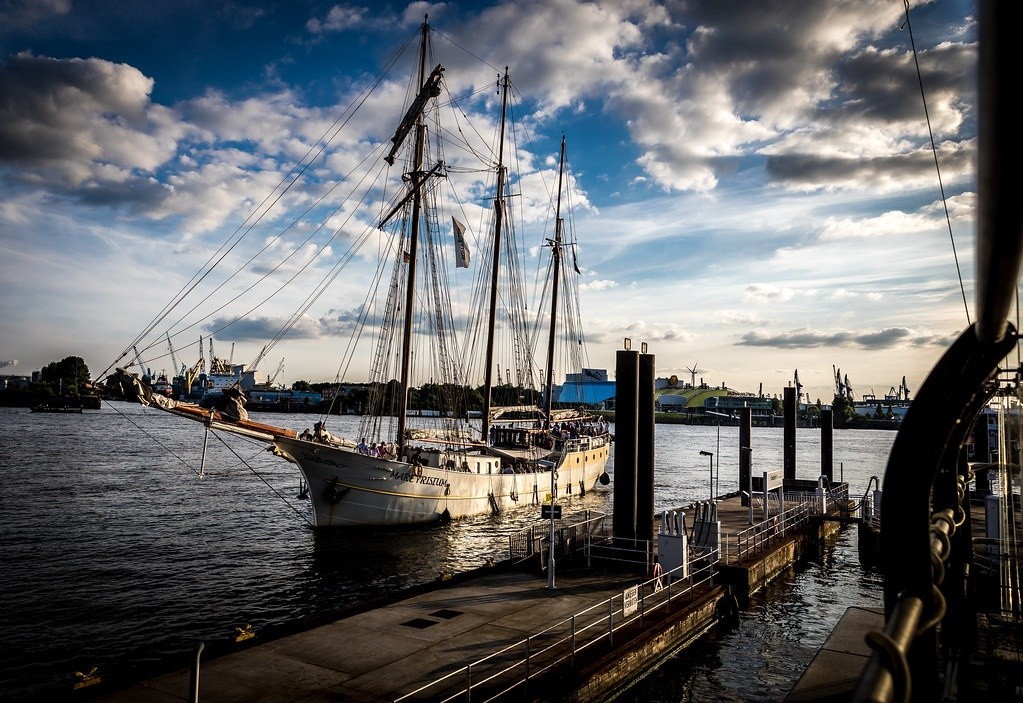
[452,216,471,268]
[572,244,580,274]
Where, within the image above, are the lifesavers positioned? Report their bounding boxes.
[652,563,664,591]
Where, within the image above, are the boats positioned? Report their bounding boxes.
[27,403,83,414]
[967,399,1023,459]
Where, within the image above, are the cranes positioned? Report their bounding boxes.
[229,342,235,364]
[199,335,205,374]
[209,338,216,367]
[132,346,148,376]
[166,331,186,376]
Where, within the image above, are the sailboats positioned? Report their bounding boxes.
[83,13,616,539]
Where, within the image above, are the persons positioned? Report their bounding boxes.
[299,421,332,445]
[601,401,605,411]
[490,406,611,474]
[354,438,396,460]
